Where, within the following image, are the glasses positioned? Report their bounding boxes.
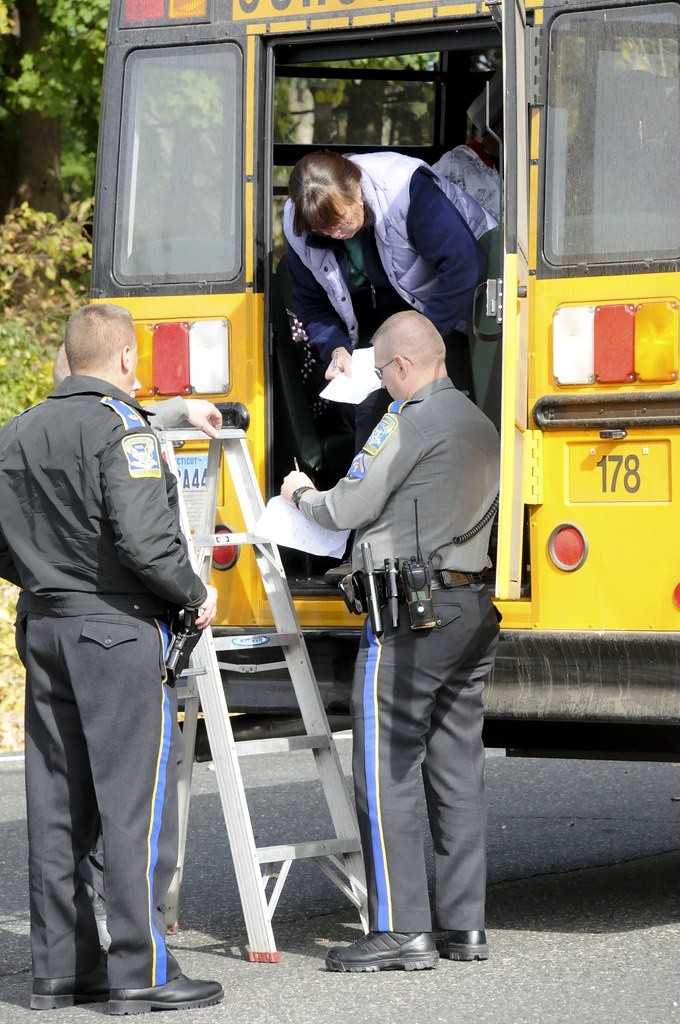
[316,204,355,234]
[374,357,414,380]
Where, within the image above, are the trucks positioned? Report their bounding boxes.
[90,0,680,755]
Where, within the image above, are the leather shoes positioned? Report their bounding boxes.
[326,932,439,972]
[30,946,110,1009]
[435,930,489,961]
[107,973,224,1015]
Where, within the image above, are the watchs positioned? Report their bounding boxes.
[292,486,315,510]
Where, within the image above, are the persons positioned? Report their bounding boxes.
[282,151,499,589]
[431,109,503,412]
[1,305,225,1016]
[280,309,504,973]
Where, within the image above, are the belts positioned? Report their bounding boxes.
[429,570,483,590]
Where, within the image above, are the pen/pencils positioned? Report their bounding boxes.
[331,350,339,381]
[294,456,300,472]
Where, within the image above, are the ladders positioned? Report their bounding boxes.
[146,426,372,965]
